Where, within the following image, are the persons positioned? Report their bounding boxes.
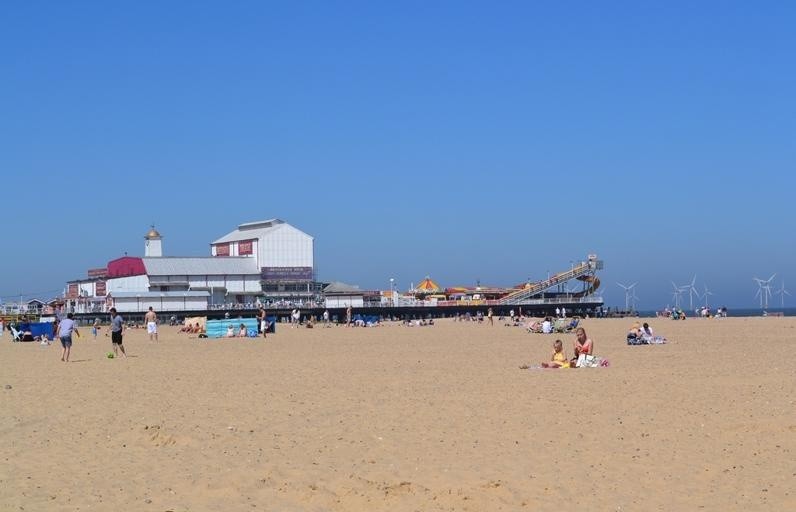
[570,326,593,368]
[662,306,686,320]
[105,308,128,360]
[56,312,80,362]
[640,323,653,343]
[694,305,727,319]
[1,306,639,346]
[541,339,569,368]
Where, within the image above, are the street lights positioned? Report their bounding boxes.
[388,277,395,306]
